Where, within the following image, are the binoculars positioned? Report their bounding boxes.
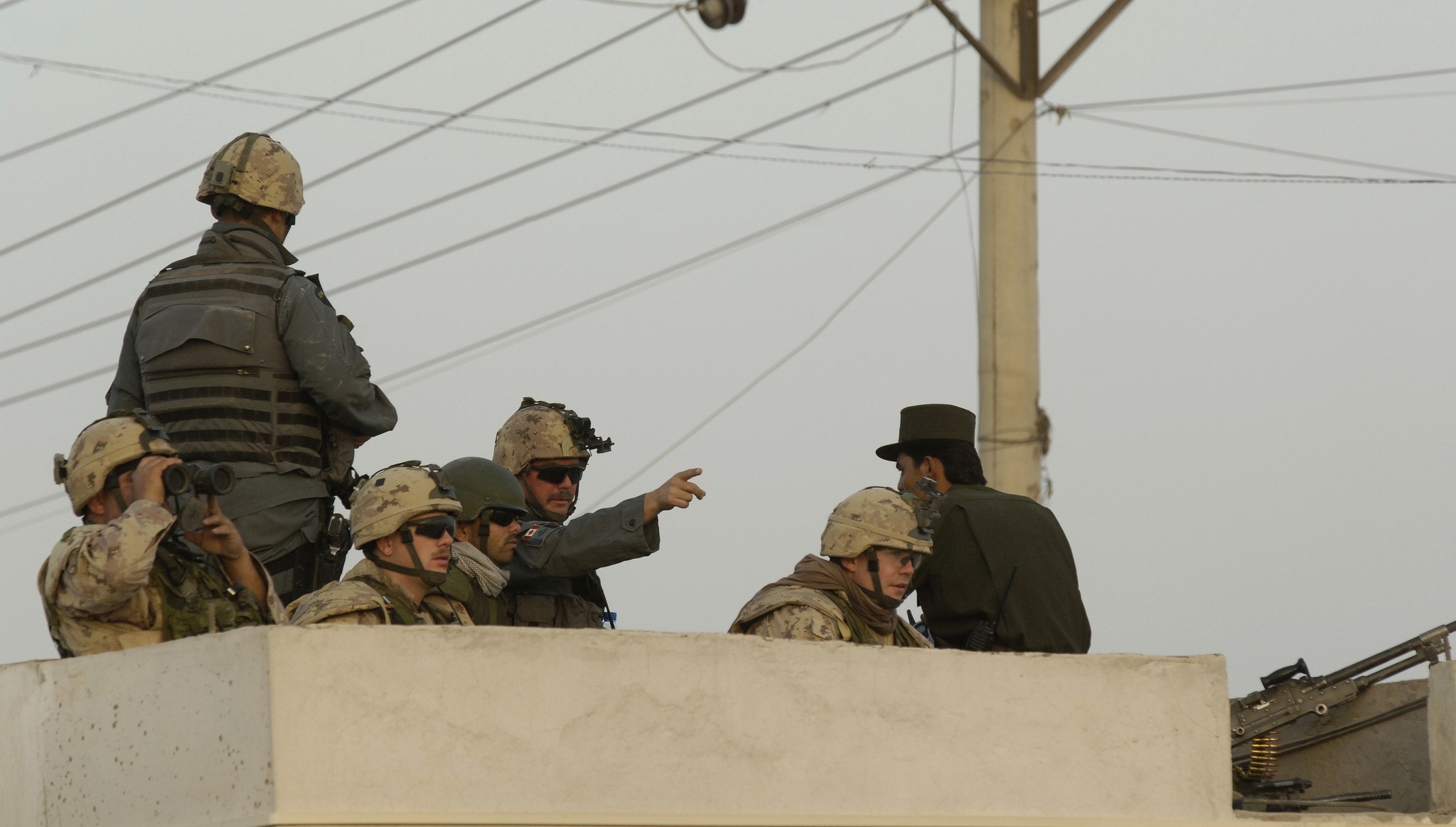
[161,463,236,497]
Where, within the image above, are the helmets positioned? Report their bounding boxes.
[347,460,463,550]
[819,476,949,560]
[492,396,616,475]
[436,456,529,525]
[195,131,305,214]
[53,407,180,518]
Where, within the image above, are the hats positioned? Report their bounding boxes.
[875,403,977,463]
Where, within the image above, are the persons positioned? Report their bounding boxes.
[731,486,935,650]
[103,131,400,608]
[425,456,530,626]
[37,406,291,660]
[491,397,706,632]
[876,403,1092,653]
[286,460,478,628]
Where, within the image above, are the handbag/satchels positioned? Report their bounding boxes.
[317,507,355,592]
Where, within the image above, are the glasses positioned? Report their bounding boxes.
[523,463,585,485]
[477,510,521,528]
[403,515,457,539]
[868,544,926,570]
